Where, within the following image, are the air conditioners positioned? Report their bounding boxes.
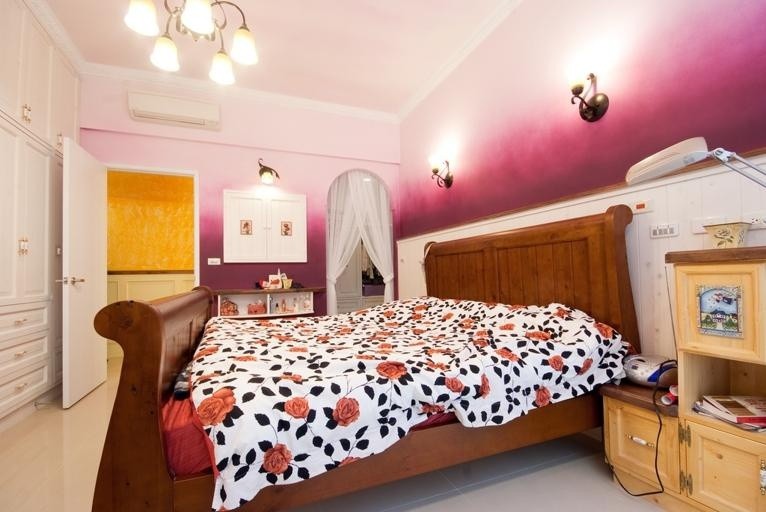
[126,90,222,132]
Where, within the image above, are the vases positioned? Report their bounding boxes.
[703,221,752,249]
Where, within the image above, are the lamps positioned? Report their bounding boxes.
[122,1,259,87]
[624,135,766,188]
[429,155,454,189]
[250,158,282,188]
[564,70,611,122]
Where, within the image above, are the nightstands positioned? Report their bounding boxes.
[594,380,679,511]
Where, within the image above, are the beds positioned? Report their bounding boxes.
[88,204,642,511]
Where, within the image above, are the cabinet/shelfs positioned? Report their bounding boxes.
[0,0,53,158]
[337,230,362,297]
[53,42,83,160]
[0,122,47,304]
[662,245,766,512]
[0,305,51,420]
[211,286,326,320]
[52,306,63,389]
[362,295,383,308]
[48,149,63,305]
[337,297,362,314]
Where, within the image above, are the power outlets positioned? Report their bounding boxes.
[742,210,766,231]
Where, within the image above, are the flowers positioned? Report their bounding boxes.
[715,226,735,249]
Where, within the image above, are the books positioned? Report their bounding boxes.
[693,401,765,432]
[702,394,766,424]
